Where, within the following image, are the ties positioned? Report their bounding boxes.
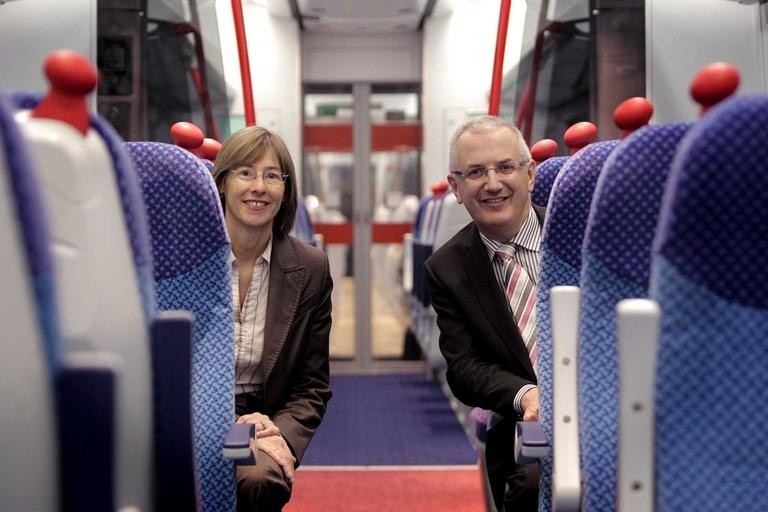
[496,242,537,379]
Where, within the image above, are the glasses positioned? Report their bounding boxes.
[451,160,530,183]
[229,169,289,185]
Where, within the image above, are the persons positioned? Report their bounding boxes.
[420,114,555,511]
[210,124,334,512]
